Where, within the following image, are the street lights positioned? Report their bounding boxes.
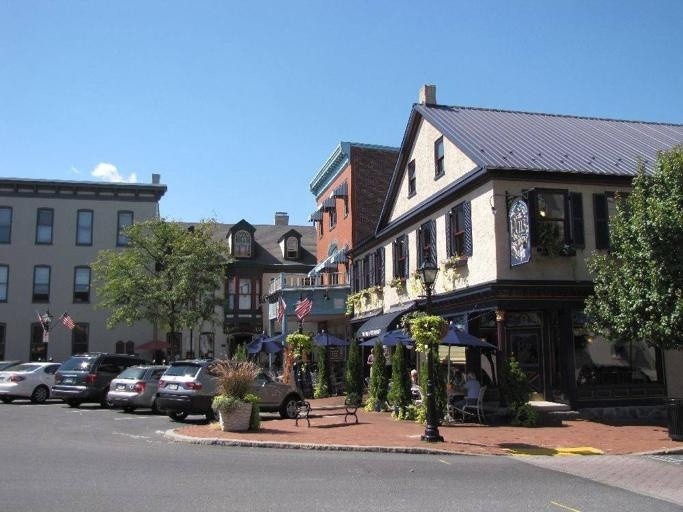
[417,256,442,444]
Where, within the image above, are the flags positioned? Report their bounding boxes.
[58,312,75,330]
[294,298,312,322]
[277,296,286,322]
[41,330,51,344]
[37,310,53,323]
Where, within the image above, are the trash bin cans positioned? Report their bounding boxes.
[667,398,683,440]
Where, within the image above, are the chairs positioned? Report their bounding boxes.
[462,386,487,423]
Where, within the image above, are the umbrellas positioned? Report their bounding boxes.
[244,320,499,356]
[135,340,172,350]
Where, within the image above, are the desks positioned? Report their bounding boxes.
[448,392,465,395]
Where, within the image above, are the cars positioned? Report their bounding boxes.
[0,359,24,371]
[0,362,61,403]
[107,365,171,414]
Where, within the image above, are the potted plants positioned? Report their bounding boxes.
[211,360,262,432]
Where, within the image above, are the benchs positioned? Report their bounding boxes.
[294,400,358,427]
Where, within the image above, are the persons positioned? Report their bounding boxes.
[332,348,482,423]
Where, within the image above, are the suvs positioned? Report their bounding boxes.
[51,352,153,408]
[159,359,303,421]
[586,365,651,383]
